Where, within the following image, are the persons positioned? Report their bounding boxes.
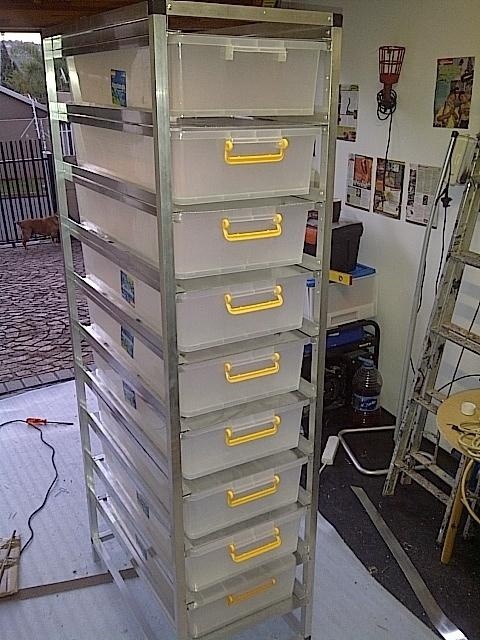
[436,92,471,128]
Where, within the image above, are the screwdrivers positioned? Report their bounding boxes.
[26,417,74,426]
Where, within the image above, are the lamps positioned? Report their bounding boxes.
[378,44,406,104]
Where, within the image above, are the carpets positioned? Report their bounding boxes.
[0,379,442,640]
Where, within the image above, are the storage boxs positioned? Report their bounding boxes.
[70,125,315,206]
[82,298,302,413]
[304,264,378,329]
[108,496,298,639]
[304,218,364,274]
[73,180,308,277]
[95,400,308,540]
[66,35,327,119]
[78,244,304,352]
[100,441,301,590]
[93,352,302,481]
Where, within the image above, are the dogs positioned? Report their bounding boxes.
[16,215,60,251]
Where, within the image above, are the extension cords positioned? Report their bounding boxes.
[321,436,339,465]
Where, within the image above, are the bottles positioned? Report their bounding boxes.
[352,355,383,431]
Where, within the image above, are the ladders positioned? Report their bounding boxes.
[381,130,480,544]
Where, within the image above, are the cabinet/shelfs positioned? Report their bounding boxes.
[299,320,380,433]
[43,0,343,638]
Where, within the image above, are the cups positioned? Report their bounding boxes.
[333,197,342,224]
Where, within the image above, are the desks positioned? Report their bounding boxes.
[436,388,480,564]
